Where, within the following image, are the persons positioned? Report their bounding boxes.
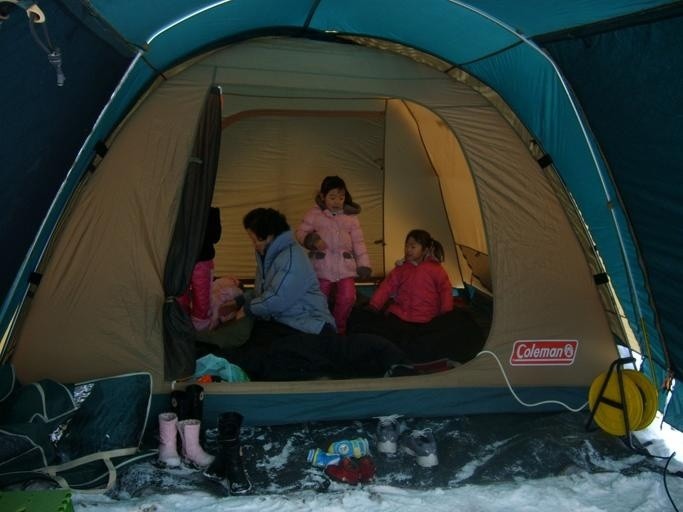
[295,175,371,337]
[234,206,338,373]
[178,244,216,331]
[368,230,454,337]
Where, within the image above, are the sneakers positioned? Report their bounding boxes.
[377,416,402,454]
[308,437,376,486]
[400,429,439,468]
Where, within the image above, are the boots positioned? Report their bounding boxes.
[158,384,252,495]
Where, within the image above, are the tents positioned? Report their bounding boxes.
[1,0,682,427]
